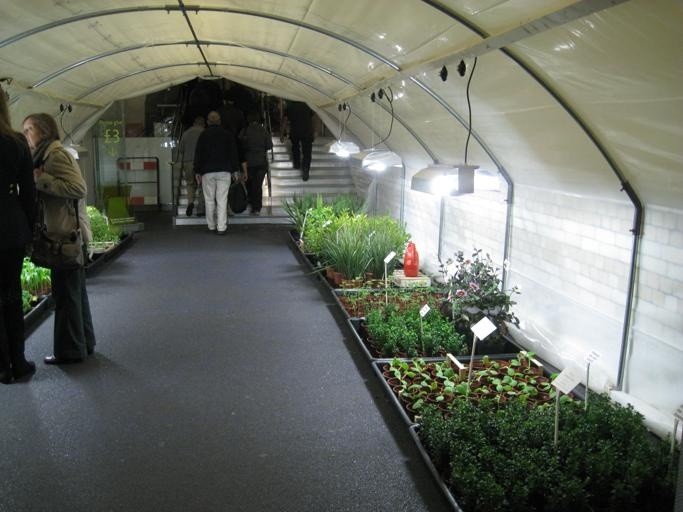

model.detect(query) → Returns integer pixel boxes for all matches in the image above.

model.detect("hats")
[208,111,221,122]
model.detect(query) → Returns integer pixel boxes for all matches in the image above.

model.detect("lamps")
[328,100,359,161]
[413,57,484,201]
[361,84,411,174]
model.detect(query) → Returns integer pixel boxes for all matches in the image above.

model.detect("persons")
[0,82,38,384]
[185,80,258,133]
[191,111,249,236]
[20,113,97,366]
[279,102,315,181]
[176,116,206,217]
[237,108,274,214]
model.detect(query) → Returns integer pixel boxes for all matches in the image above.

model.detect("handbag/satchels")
[29,197,84,270]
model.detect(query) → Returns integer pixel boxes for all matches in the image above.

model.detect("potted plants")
[317,215,409,289]
[357,300,469,358]
[337,275,444,316]
[381,350,575,421]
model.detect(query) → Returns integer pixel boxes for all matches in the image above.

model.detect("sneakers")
[251,209,259,214]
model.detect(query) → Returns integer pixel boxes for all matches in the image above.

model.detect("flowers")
[440,254,526,351]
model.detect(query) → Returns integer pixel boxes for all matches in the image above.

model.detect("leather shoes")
[197,212,206,217]
[15,360,35,377]
[185,202,194,216]
[216,228,228,235]
[44,355,83,363]
[208,229,217,233]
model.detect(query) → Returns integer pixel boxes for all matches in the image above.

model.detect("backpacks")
[229,181,249,214]
